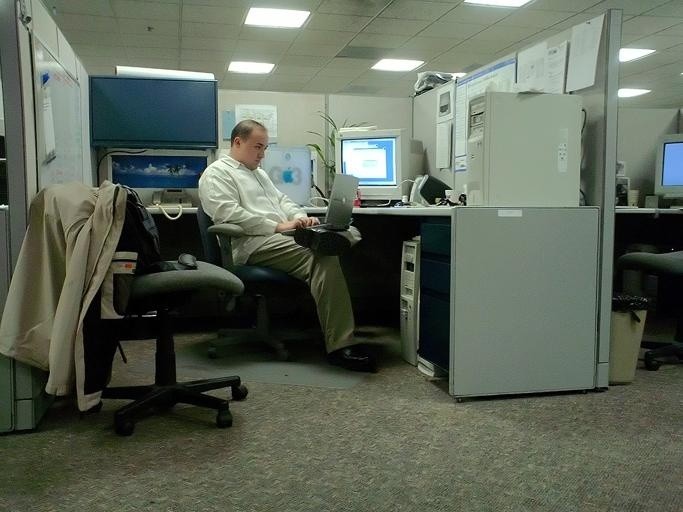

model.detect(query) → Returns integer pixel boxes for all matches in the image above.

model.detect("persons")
[199,120,382,371]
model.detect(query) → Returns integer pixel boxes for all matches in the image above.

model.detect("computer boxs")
[399,238,421,367]
[260,147,313,208]
[466,93,583,206]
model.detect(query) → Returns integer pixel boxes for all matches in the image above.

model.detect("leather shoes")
[326,348,371,371]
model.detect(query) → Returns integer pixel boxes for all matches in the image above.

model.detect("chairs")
[617,241,682,371]
[80,184,248,438]
[193,198,312,363]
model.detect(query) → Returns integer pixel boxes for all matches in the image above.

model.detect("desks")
[72,200,601,403]
[614,207,682,214]
[122,195,295,252]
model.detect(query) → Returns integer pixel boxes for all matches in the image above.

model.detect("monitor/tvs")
[334,129,425,207]
[654,133,683,208]
[89,74,219,149]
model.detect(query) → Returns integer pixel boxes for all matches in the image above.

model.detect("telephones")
[150,186,193,220]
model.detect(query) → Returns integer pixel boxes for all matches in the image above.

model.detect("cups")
[445,190,458,205]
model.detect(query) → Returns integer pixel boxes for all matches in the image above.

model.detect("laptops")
[282,173,360,236]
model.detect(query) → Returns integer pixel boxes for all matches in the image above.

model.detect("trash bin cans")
[608,296,651,385]
